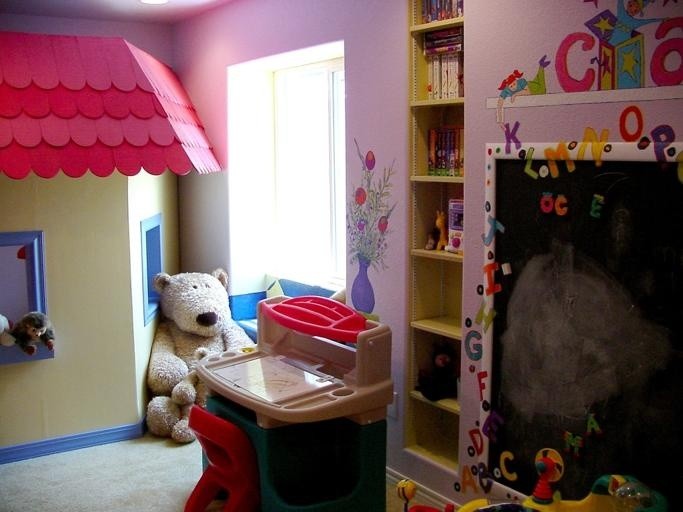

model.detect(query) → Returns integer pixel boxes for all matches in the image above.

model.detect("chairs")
[186,403,261,510]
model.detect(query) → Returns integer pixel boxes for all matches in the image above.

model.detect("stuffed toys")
[416,342,458,403]
[0,314,16,347]
[170,346,219,444]
[145,268,256,436]
[8,310,53,356]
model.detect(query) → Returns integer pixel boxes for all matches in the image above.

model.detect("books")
[417,0,463,177]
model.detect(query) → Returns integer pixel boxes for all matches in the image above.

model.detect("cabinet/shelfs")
[402,1,465,482]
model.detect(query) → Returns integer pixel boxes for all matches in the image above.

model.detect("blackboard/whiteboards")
[476,143,683,512]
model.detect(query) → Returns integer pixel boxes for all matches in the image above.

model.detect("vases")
[350,254,375,314]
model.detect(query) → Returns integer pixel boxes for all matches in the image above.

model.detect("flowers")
[344,137,398,273]
